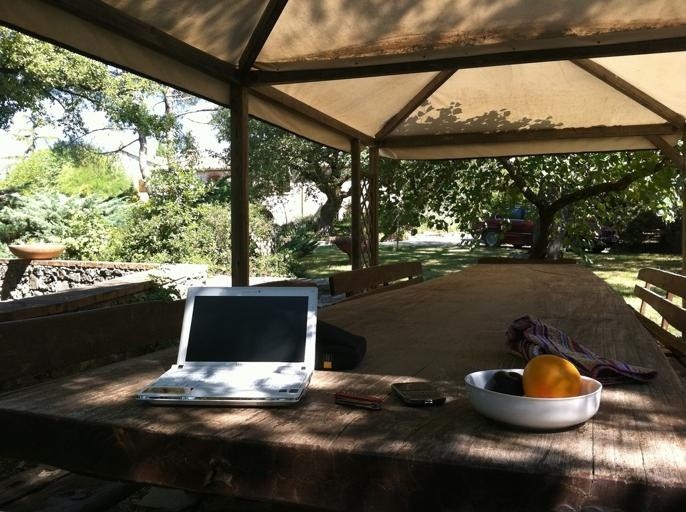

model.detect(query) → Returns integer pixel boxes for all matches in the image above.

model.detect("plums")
[484,370,524,396]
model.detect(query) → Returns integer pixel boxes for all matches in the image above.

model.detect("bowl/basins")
[464,368,603,430]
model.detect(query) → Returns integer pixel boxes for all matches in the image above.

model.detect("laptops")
[136,286,318,406]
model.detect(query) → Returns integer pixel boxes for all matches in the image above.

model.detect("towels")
[505,312,657,387]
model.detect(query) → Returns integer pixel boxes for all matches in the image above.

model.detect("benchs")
[328,261,425,307]
[0,281,317,511]
[627,268,686,393]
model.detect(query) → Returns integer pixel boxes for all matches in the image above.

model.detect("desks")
[0,258,686,512]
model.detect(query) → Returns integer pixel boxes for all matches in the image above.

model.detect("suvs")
[473,201,622,254]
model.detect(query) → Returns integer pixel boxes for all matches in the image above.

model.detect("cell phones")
[391,382,444,406]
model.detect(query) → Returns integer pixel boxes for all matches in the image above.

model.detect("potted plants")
[0,208,66,260]
